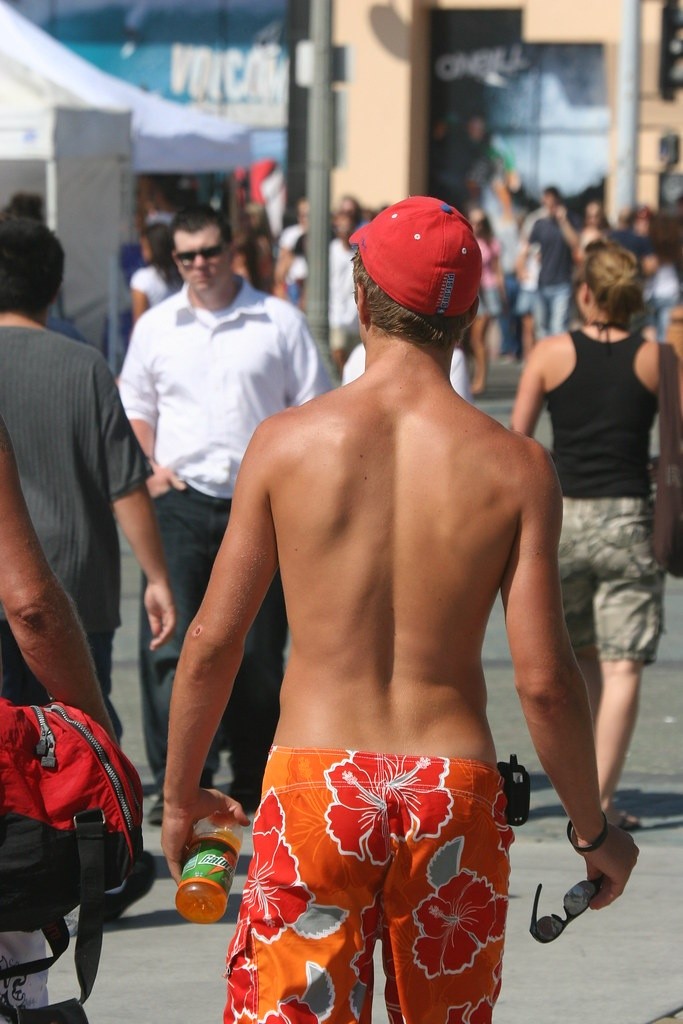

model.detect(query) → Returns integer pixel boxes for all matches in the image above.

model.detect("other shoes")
[612,811,641,830]
[104,850,155,921]
[149,779,212,825]
[229,779,260,811]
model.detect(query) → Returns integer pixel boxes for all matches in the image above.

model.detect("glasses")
[176,245,223,260]
[529,873,606,944]
[582,233,608,253]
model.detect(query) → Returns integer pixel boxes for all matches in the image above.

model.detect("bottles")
[175,811,244,924]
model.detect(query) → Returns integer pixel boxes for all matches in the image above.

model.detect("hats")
[349,195,482,317]
[468,207,486,223]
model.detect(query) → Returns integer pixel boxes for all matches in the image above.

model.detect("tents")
[2,0,254,375]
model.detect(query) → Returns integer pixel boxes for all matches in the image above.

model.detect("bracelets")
[567,812,608,851]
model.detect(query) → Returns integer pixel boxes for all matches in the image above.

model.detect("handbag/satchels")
[649,342,683,577]
[0,696,143,934]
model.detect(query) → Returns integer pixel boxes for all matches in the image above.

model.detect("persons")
[0,173,683,1024]
[159,197,640,1024]
[509,247,683,832]
